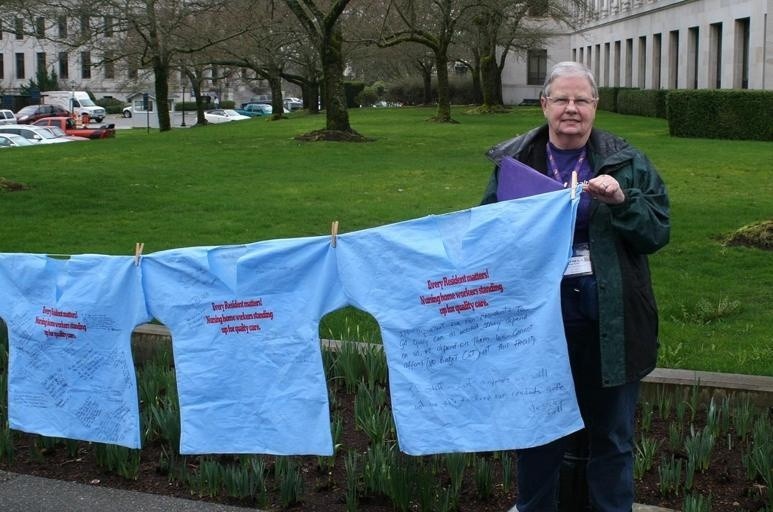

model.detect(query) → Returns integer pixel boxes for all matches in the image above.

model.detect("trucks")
[39,89,107,124]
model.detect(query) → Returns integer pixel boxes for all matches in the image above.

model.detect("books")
[495,154,563,204]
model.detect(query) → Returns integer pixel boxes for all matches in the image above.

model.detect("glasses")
[546,96,596,108]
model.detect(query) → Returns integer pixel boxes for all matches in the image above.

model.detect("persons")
[478,60,670,512]
[213,96,218,110]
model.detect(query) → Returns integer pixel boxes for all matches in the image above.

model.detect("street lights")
[179,78,189,126]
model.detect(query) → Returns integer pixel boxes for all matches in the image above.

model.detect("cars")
[0,103,116,149]
[231,97,305,117]
[123,105,144,117]
[359,100,404,108]
[203,107,251,123]
